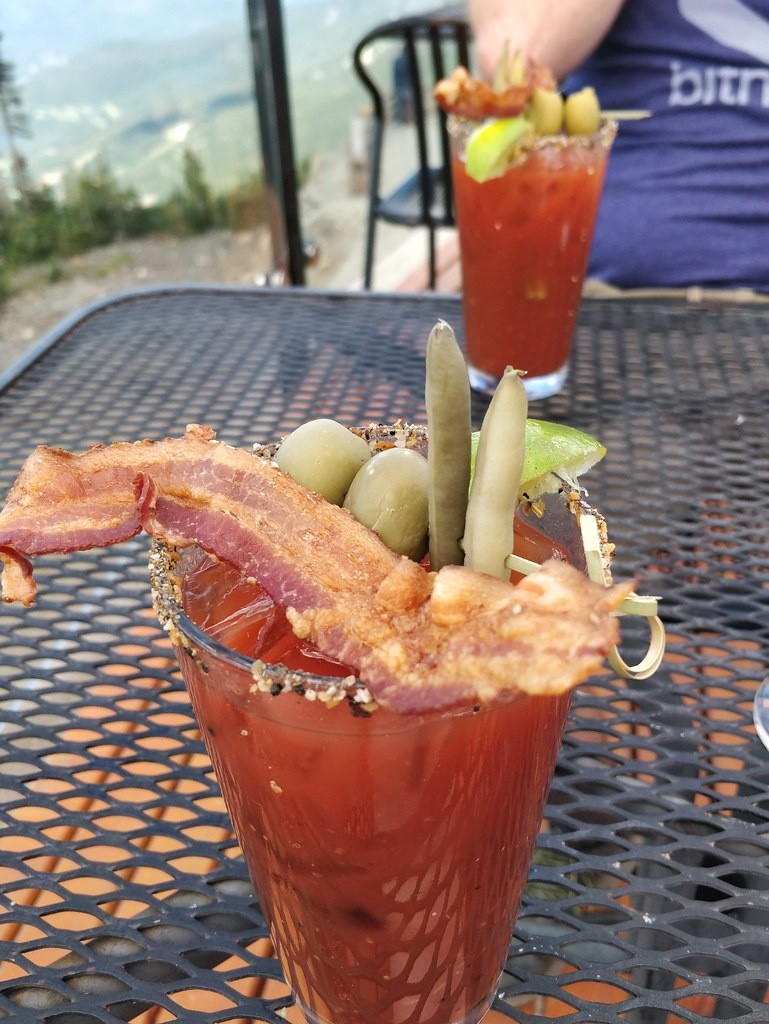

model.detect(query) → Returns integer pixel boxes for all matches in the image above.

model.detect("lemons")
[466,416,607,505]
[464,117,535,184]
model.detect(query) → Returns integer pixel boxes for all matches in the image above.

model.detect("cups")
[148,439,593,1024]
[446,113,617,401]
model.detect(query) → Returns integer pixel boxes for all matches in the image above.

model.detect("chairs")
[358,15,473,291]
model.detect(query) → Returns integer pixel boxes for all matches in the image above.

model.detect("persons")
[464,0,769,301]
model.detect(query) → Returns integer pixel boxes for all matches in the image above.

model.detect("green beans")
[425,318,528,580]
[494,40,524,92]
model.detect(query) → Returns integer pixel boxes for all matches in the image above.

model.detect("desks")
[0,287,769,1024]
